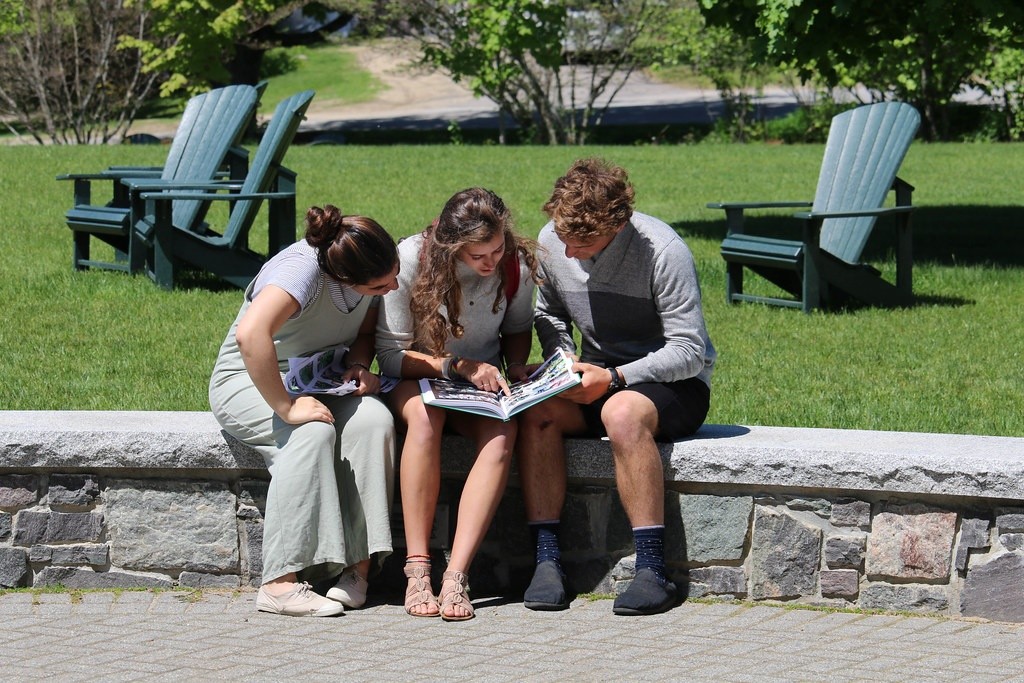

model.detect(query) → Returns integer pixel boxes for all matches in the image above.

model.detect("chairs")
[706,102,922,311]
[56,81,315,291]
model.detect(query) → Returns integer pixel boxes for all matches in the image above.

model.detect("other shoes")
[612,568,679,614]
[523,561,568,610]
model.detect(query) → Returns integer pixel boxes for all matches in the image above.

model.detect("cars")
[523,0,629,66]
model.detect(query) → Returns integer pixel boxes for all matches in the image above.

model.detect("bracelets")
[508,360,525,368]
[448,356,464,380]
[442,357,454,380]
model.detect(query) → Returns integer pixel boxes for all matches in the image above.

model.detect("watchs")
[607,368,624,393]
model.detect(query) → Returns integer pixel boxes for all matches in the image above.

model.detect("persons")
[375,189,542,622]
[517,157,717,615]
[208,204,400,617]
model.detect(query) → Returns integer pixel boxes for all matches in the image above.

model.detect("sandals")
[436,572,474,620]
[404,564,442,616]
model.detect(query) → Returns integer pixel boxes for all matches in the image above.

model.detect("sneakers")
[326,565,368,608]
[256,581,344,617]
